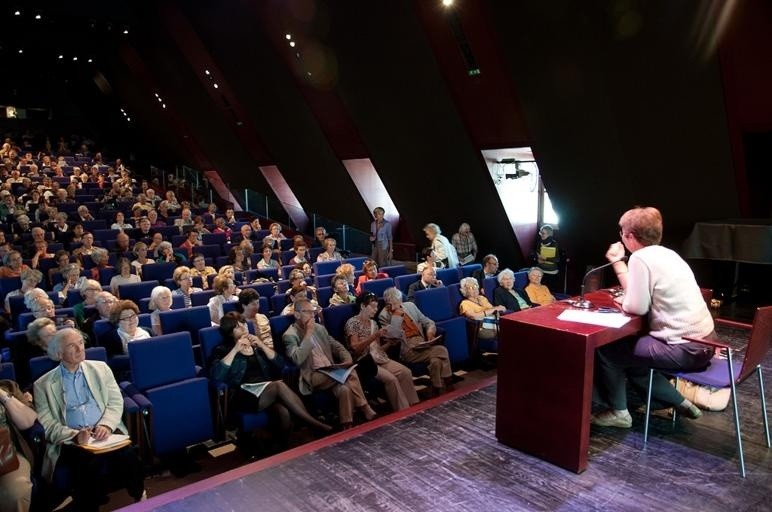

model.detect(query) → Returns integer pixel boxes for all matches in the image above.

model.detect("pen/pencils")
[79,424,94,434]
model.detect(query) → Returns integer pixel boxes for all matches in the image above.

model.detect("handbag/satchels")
[435,257,449,268]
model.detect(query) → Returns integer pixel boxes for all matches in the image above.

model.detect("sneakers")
[669,405,702,419]
[592,411,632,428]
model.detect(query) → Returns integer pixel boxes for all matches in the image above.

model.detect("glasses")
[119,314,136,321]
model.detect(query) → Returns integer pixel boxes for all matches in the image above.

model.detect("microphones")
[572,255,628,308]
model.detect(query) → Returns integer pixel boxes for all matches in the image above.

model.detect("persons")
[616,270,628,275]
[589,207,718,428]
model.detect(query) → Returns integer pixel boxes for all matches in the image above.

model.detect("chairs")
[0,144,563,512]
[639,305,771,481]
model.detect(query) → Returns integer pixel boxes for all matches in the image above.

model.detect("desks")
[496,286,718,476]
